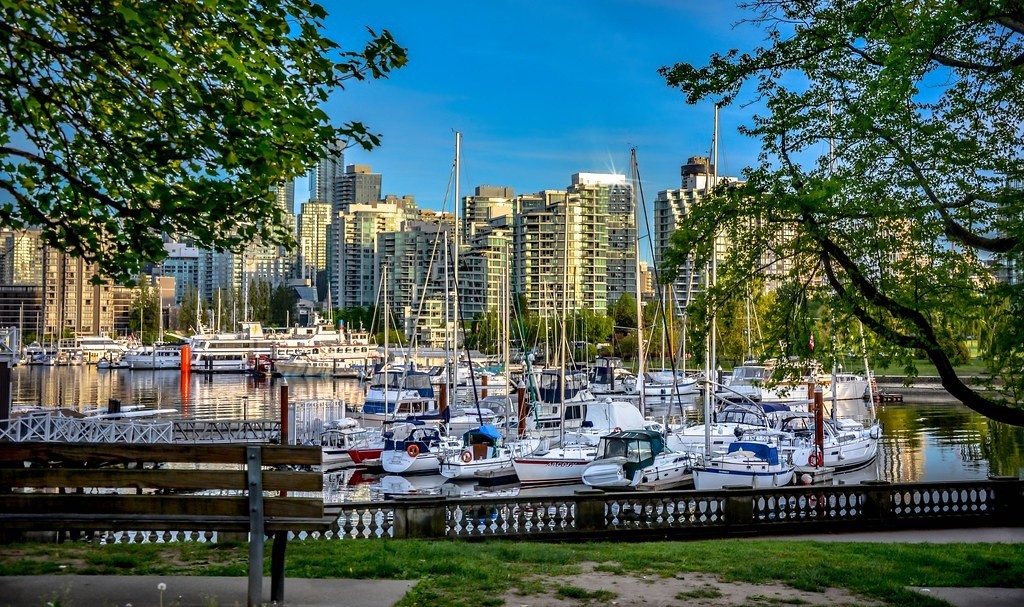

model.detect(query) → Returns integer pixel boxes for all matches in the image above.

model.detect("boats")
[21,265,392,380]
[368,475,460,498]
[582,428,700,490]
[307,416,382,474]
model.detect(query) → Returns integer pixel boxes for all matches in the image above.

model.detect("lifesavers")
[408,445,420,457]
[614,427,623,432]
[807,454,821,467]
[461,450,472,463]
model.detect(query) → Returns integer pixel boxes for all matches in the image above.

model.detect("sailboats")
[304,96,883,489]
